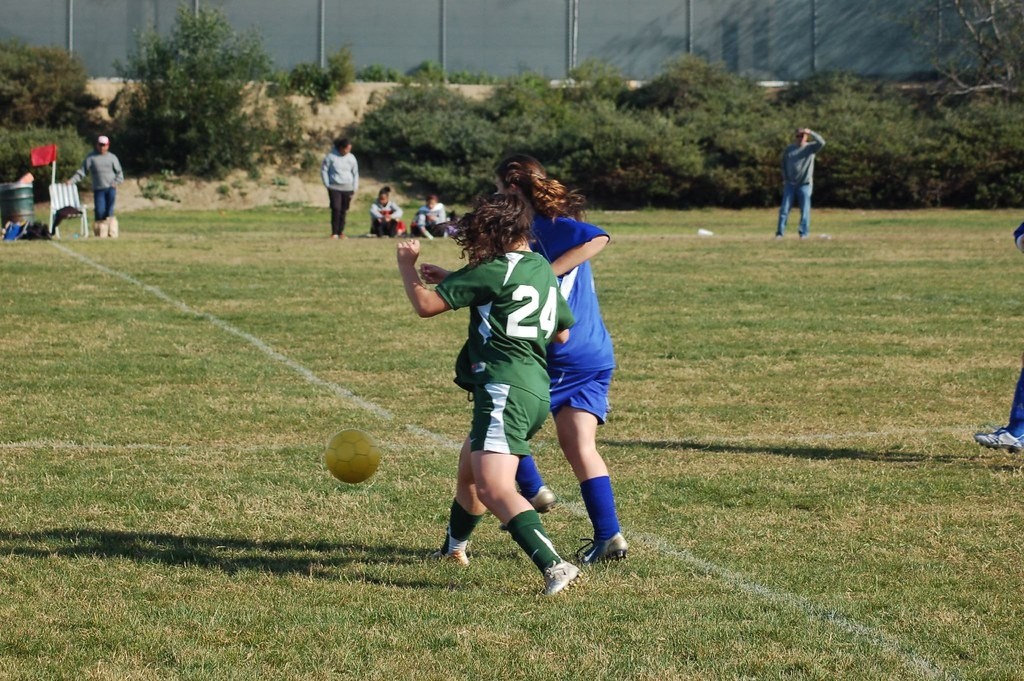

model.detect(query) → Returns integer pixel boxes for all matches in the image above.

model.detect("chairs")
[49,182,89,238]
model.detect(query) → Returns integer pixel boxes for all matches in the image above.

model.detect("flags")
[30,144,56,166]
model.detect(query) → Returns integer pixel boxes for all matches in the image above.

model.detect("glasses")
[98,144,107,147]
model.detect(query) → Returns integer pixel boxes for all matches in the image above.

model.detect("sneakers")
[433,550,469,565]
[974,427,1024,451]
[542,559,579,595]
[499,487,558,531]
[575,532,629,566]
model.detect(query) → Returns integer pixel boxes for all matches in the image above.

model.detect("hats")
[98,135,109,145]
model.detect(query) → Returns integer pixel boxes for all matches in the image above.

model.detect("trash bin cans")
[0,183,35,232]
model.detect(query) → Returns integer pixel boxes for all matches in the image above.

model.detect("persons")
[420,153,629,564]
[973,222,1024,452]
[410,196,448,239]
[362,186,403,238]
[320,138,359,241]
[775,127,826,240]
[396,194,579,596]
[64,135,124,221]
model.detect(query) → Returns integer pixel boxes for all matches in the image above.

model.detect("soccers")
[323,428,383,484]
